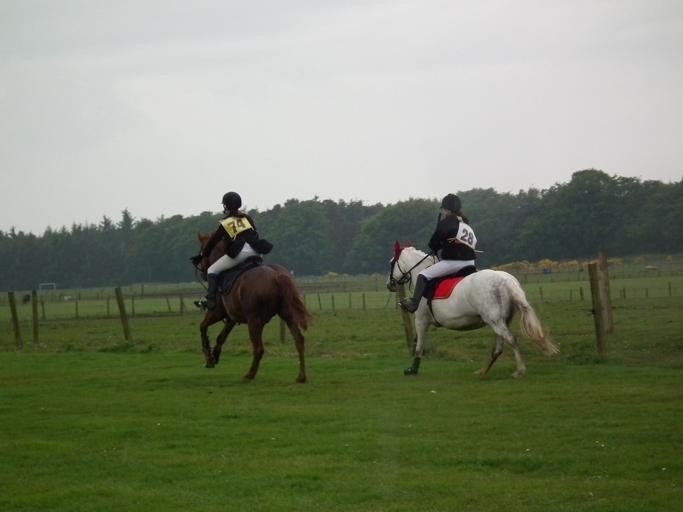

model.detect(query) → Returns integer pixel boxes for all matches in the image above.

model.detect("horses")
[198,233,315,383]
[386,240,561,380]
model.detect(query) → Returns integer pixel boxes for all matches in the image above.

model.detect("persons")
[397,194,478,312]
[194,192,274,309]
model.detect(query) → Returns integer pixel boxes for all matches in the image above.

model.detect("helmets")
[441,194,462,212]
[222,191,242,208]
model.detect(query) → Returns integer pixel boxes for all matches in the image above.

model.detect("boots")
[400,274,427,313]
[194,273,217,309]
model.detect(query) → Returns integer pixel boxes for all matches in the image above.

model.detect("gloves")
[190,255,203,265]
